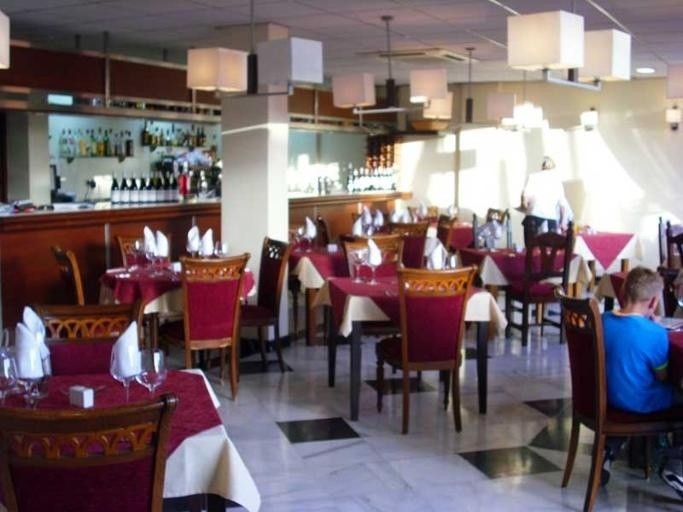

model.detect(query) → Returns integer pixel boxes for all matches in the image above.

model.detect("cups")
[109,348,140,407]
[135,346,168,404]
[14,346,44,414]
[0,345,17,410]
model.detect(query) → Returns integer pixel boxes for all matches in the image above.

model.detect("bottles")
[147,169,178,204]
[119,170,129,205]
[195,170,209,203]
[56,127,134,157]
[177,161,191,203]
[345,161,397,193]
[140,118,207,148]
[127,169,138,204]
[137,170,147,203]
[110,171,119,205]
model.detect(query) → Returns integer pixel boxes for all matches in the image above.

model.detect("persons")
[592,266,682,495]
[519,155,569,251]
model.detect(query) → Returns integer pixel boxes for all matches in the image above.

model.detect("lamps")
[246,0,257,94]
[506,2,631,93]
[332,14,448,113]
[382,15,395,106]
[186,0,324,99]
[464,45,473,122]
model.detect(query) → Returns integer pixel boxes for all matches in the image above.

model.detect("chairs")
[504,219,574,346]
[48,247,94,306]
[373,264,477,434]
[315,216,333,248]
[436,215,458,252]
[338,232,406,374]
[555,288,683,512]
[166,254,250,402]
[0,392,180,512]
[240,236,290,379]
[480,206,510,250]
[27,300,144,375]
[386,221,429,270]
[116,233,147,268]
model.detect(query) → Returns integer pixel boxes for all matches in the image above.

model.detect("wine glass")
[346,246,380,286]
[676,284,682,329]
[424,249,464,272]
[290,225,314,253]
[132,239,168,277]
[184,238,229,261]
[360,209,384,237]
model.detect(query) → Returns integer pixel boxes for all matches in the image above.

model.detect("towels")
[157,230,169,257]
[23,306,52,374]
[200,227,214,256]
[187,224,200,252]
[143,226,156,256]
[109,319,142,379]
[15,322,45,380]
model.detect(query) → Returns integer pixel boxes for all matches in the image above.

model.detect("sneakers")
[602,446,612,484]
[660,468,682,495]
